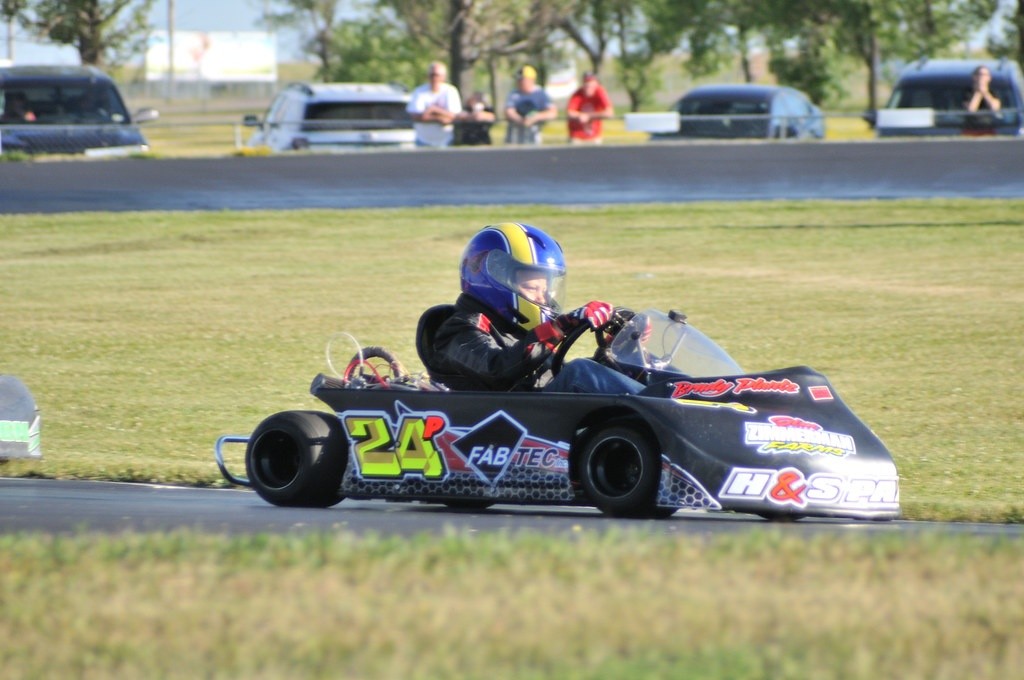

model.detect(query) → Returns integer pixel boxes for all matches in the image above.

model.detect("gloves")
[555,300,613,336]
[605,316,652,344]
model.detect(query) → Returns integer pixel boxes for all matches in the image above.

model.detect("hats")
[517,65,536,79]
[583,72,596,82]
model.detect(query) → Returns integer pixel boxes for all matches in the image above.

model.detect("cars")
[651,85,824,140]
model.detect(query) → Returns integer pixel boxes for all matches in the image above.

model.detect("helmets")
[460,222,567,340]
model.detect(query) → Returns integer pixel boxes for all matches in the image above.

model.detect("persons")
[416,223,694,395]
[9,92,38,121]
[566,72,614,144]
[506,66,556,143]
[407,61,497,147]
[960,64,1003,137]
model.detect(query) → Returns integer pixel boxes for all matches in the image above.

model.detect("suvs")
[1,62,158,158]
[862,54,1024,137]
[243,80,418,152]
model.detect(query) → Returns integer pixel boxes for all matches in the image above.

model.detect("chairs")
[416,304,537,393]
